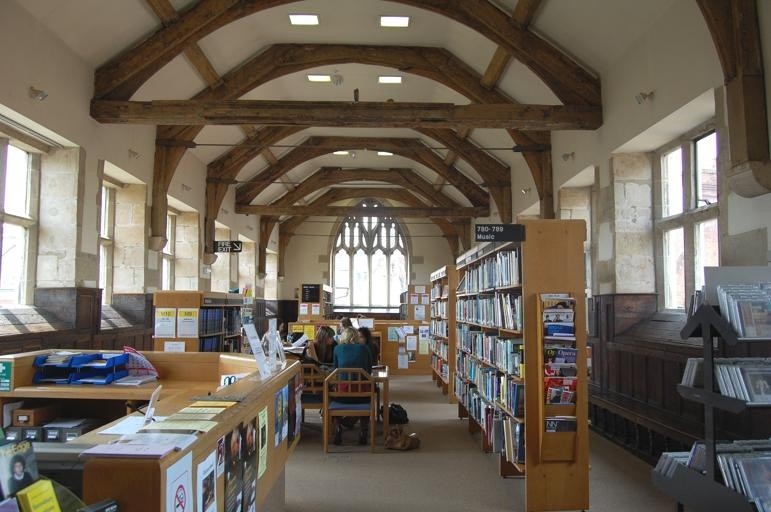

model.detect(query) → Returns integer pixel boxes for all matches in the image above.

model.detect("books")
[114,375,157,385]
[40,351,107,383]
[427,246,577,461]
[655,284,771,511]
[0,426,120,511]
[198,306,242,352]
[399,291,409,320]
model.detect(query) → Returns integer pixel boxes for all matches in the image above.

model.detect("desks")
[369,363,392,445]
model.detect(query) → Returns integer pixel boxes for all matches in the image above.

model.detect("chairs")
[282,340,379,455]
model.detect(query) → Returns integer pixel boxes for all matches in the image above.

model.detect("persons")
[357,326,381,366]
[300,325,338,367]
[330,326,375,445]
[339,316,354,331]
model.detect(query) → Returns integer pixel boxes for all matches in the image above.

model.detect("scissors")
[223,376,236,386]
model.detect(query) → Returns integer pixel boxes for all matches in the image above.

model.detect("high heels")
[332,425,344,447]
[357,431,367,445]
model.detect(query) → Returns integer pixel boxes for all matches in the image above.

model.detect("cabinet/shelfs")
[431,264,454,404]
[399,282,431,322]
[297,283,334,320]
[152,288,245,353]
[651,300,771,512]
[455,218,592,512]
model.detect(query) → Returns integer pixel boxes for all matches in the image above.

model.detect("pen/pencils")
[125,403,156,421]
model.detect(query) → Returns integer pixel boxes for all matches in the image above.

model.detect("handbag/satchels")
[380,403,411,423]
[383,426,421,451]
